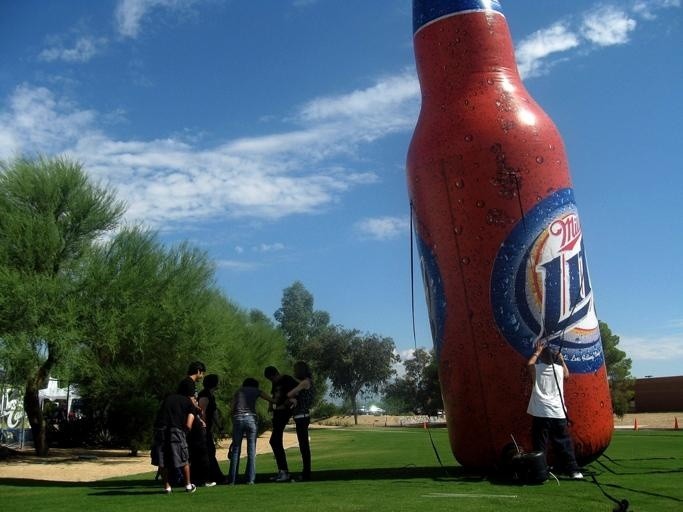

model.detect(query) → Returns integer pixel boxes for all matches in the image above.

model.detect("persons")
[263,364,301,482]
[41,399,85,427]
[177,361,217,488]
[286,360,316,481]
[222,377,277,486]
[196,374,230,484]
[150,394,202,494]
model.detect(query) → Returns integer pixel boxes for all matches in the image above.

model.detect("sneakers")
[205,482,216,487]
[186,484,196,493]
[165,489,173,495]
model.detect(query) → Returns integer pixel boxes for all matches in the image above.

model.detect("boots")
[270,470,290,482]
